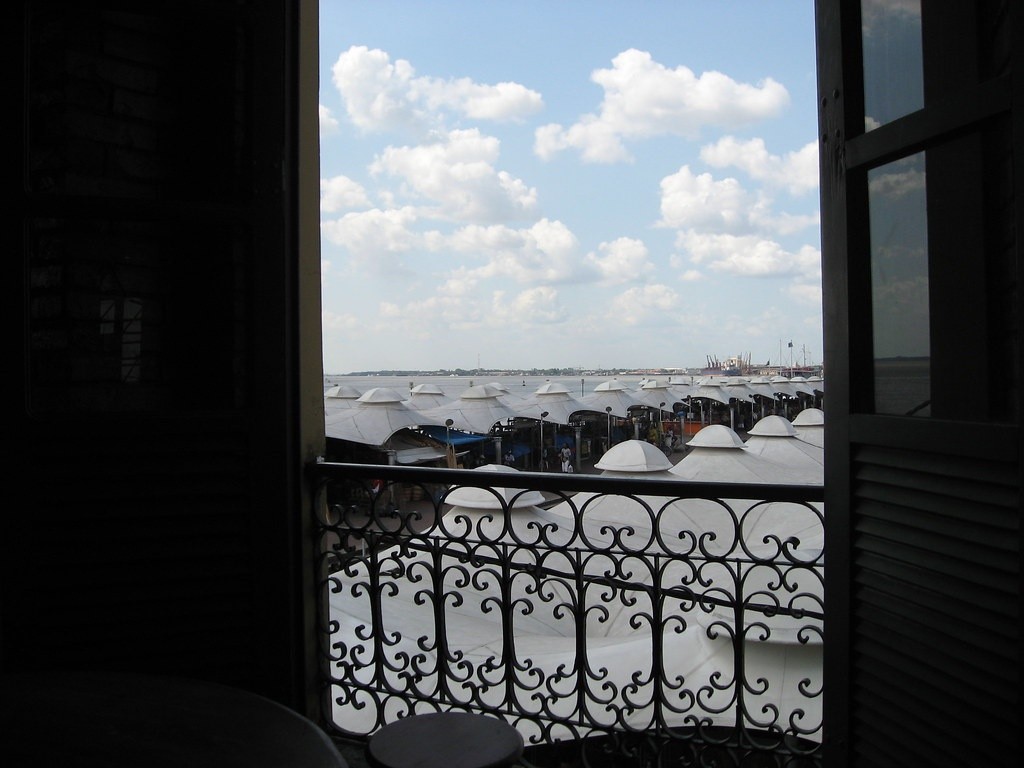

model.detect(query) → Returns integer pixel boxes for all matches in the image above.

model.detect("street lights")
[659,402,665,447]
[409,381,413,397]
[749,394,754,427]
[773,393,777,415]
[605,406,613,450]
[687,395,691,436]
[445,418,454,468]
[540,410,549,472]
[581,379,585,396]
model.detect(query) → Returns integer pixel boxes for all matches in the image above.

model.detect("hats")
[480,455,485,459]
[508,449,511,453]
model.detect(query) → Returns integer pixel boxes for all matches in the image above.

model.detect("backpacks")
[476,460,479,464]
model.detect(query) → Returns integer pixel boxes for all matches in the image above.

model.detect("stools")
[364,712,537,768]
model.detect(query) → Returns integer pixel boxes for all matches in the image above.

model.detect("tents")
[324,374,824,745]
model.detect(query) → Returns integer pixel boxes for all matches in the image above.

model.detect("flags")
[788,342,792,347]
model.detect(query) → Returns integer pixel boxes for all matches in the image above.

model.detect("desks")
[0,670,343,768]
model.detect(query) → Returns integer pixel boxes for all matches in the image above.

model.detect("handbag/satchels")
[568,465,573,473]
